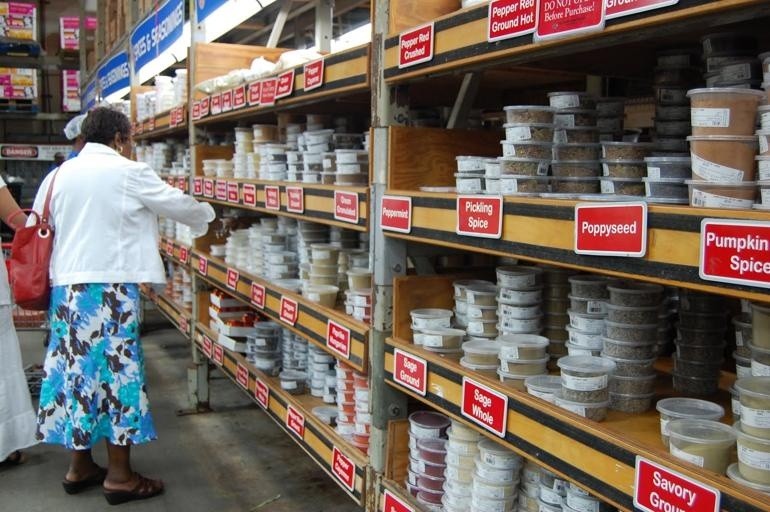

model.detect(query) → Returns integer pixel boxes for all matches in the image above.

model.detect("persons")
[19,105,251,507]
[0,167,47,471]
[34,112,95,203]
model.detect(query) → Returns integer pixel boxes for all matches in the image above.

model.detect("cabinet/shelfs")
[78,0,192,340]
[1,51,87,239]
[380,0,770,213]
[189,189,369,504]
[194,1,377,200]
[372,214,770,512]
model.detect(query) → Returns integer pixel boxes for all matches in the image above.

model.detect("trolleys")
[0,244,49,396]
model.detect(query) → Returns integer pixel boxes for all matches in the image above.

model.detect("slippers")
[63,462,107,495]
[103,471,165,506]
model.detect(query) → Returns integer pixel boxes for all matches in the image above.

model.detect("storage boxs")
[59,17,80,53]
[0,0,41,44]
[0,66,42,100]
[60,69,81,113]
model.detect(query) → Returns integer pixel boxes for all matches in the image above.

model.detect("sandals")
[0,451,24,469]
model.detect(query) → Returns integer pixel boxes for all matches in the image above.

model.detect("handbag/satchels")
[10,223,53,312]
